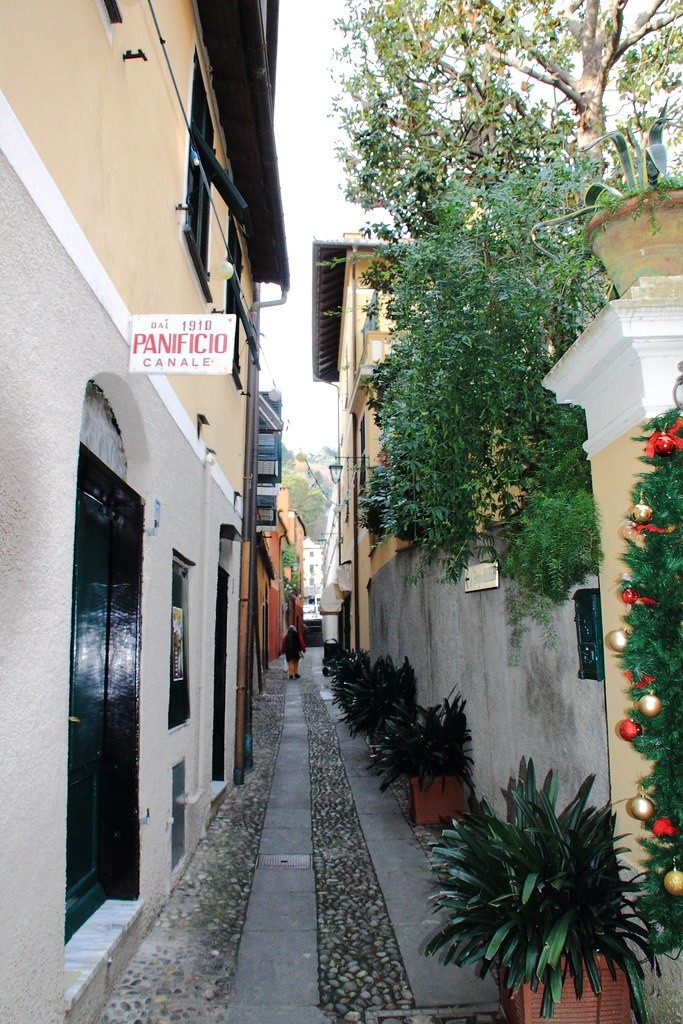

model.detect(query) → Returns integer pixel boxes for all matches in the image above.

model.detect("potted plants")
[417,753,665,1024]
[339,658,478,826]
[529,98,683,298]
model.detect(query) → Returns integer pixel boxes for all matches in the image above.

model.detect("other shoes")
[289,676,293,678]
[295,674,300,678]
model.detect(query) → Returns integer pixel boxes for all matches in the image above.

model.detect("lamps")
[240,391,251,397]
[296,448,307,462]
[329,455,370,484]
[207,262,235,280]
[191,145,199,166]
[123,49,147,62]
[269,382,281,401]
[325,497,332,507]
[333,505,339,513]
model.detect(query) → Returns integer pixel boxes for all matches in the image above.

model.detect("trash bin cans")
[324,638,337,658]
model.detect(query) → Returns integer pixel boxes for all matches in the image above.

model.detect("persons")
[282,625,306,678]
